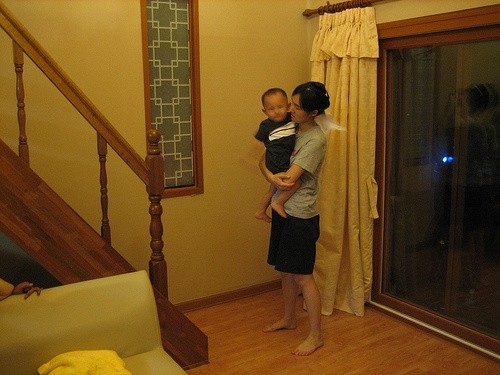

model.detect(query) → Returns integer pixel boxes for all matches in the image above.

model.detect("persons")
[254,87,301,223]
[0,278,43,300]
[259,81,331,355]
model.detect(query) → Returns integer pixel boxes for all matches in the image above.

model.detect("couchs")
[0,270,188,375]
